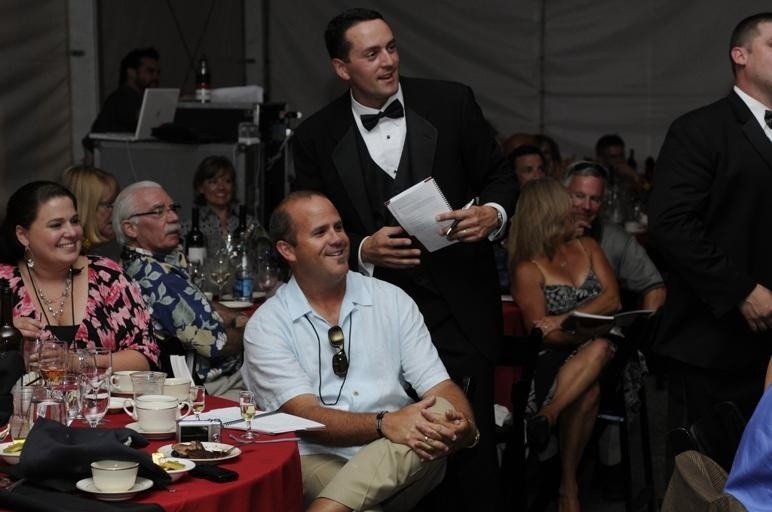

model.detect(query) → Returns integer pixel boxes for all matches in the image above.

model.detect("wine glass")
[23,338,112,430]
[190,386,206,420]
[239,391,258,440]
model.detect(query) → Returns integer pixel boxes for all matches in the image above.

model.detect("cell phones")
[189,464,239,482]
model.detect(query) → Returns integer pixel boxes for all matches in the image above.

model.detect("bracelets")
[231,311,242,327]
[93,352,98,366]
[374,410,388,436]
[465,430,480,450]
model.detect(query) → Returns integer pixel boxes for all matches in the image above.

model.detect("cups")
[108,370,192,432]
[194,58,212,102]
[185,251,279,308]
[91,459,139,491]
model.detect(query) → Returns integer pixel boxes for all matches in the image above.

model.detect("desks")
[95,138,266,227]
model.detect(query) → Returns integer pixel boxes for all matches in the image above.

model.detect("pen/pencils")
[446,199,475,236]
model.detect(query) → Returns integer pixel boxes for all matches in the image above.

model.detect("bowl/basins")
[625,222,641,230]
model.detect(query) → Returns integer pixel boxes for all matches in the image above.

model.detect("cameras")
[177,419,223,444]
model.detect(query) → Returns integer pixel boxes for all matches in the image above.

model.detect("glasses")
[328,325,347,377]
[127,204,182,221]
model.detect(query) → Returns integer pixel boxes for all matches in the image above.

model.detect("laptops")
[88,87,182,143]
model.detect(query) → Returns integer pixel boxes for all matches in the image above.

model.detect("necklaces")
[26,267,73,325]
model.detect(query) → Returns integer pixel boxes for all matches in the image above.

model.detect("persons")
[0,180,163,374]
[290,10,520,512]
[178,155,273,264]
[562,161,669,509]
[81,49,161,169]
[510,179,622,510]
[658,11,772,470]
[113,180,251,383]
[58,166,123,263]
[244,190,482,511]
[502,132,664,239]
[722,357,771,509]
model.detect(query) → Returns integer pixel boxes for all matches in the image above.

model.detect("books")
[559,309,655,334]
[384,176,463,254]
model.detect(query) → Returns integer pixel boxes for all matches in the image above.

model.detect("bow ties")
[360,98,404,132]
[765,111,772,127]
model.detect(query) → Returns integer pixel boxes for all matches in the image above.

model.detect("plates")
[0,442,21,465]
[150,441,242,481]
[76,477,154,501]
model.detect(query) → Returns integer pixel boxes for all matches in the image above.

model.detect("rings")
[544,324,548,330]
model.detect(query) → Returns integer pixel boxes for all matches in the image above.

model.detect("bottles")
[184,204,258,302]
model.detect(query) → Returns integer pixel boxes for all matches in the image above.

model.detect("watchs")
[492,206,503,227]
